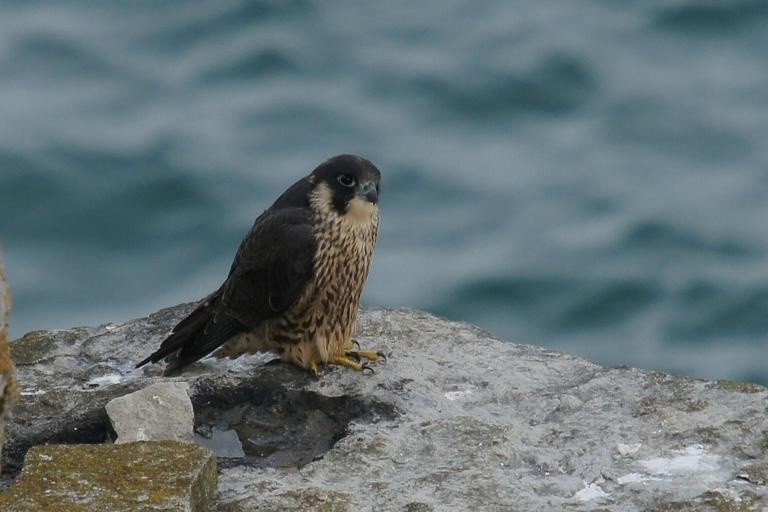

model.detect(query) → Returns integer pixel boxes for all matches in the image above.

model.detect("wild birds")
[133,153,385,382]
